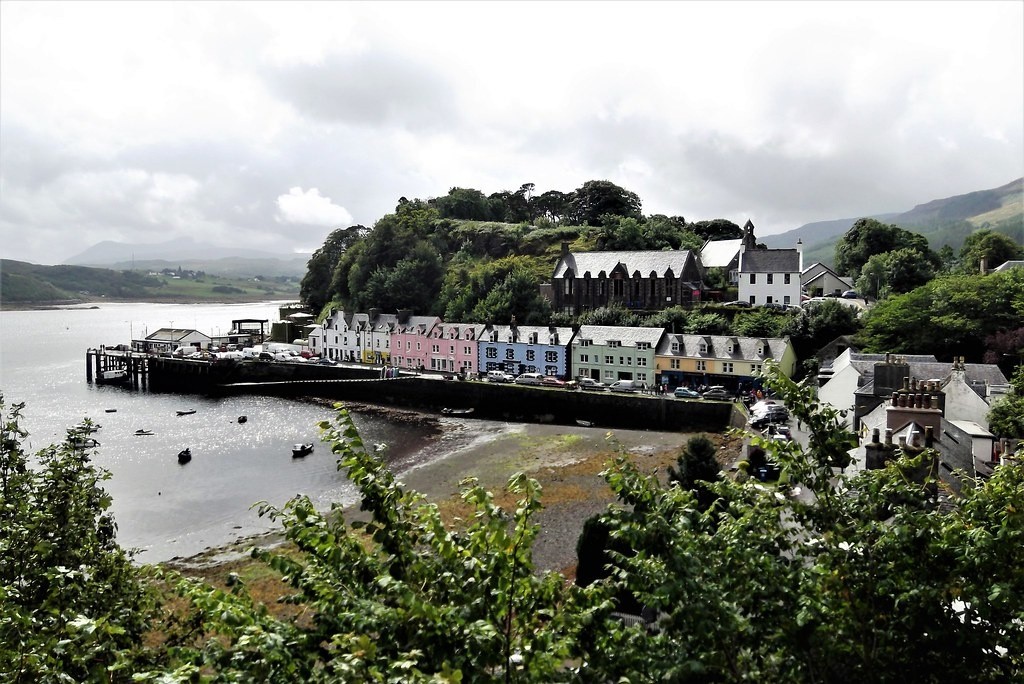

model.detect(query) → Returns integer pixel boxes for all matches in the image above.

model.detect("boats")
[178,448,191,465]
[105,408,117,413]
[135,429,154,435]
[176,408,196,416]
[292,443,314,458]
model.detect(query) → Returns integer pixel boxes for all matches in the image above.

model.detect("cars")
[320,358,337,366]
[259,349,307,364]
[674,386,700,399]
[198,344,242,361]
[543,377,566,388]
[308,357,319,364]
[301,350,313,359]
[750,400,789,430]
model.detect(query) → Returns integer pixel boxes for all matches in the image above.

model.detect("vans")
[610,380,636,393]
[515,373,544,386]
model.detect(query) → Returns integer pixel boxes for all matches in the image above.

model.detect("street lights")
[143,323,149,352]
[125,320,134,351]
[169,320,174,354]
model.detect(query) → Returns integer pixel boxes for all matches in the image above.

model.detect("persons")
[751,388,762,399]
[679,381,690,389]
[639,383,668,397]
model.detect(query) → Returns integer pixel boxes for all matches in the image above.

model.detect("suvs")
[487,371,514,382]
[580,378,605,390]
[703,389,729,401]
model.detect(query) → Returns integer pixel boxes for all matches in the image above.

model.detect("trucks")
[243,348,260,358]
[174,347,196,357]
